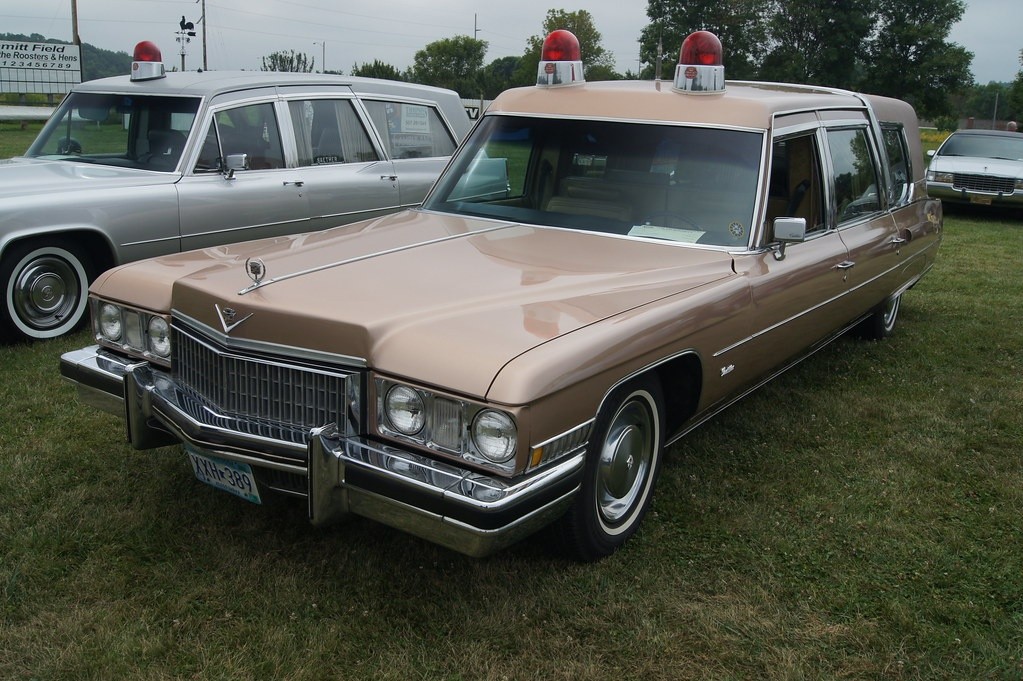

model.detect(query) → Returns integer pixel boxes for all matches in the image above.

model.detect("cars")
[926,128,1022,216]
[55,29,942,561]
[0,39,511,343]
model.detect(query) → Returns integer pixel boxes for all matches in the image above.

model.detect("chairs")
[318,127,343,162]
[223,128,266,169]
[543,168,788,244]
[148,129,187,167]
